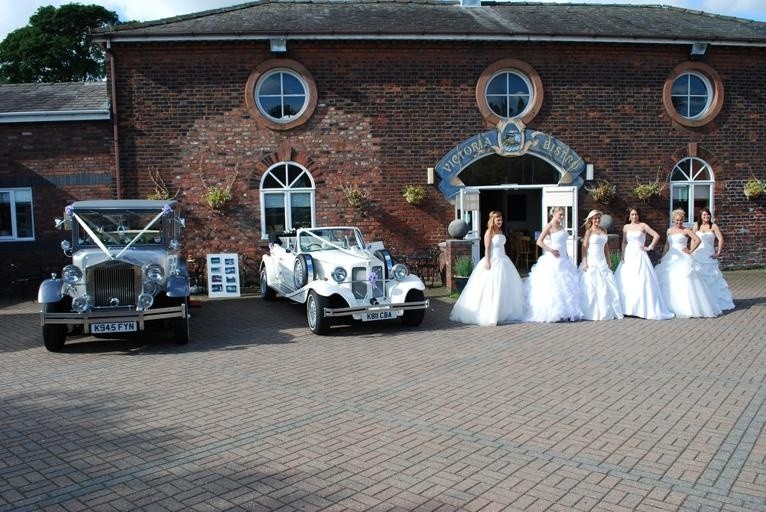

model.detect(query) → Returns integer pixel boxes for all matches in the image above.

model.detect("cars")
[37,199,195,349]
[259,225,430,336]
[0,254,54,300]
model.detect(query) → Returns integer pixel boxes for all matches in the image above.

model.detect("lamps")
[687,42,710,56]
[266,38,289,55]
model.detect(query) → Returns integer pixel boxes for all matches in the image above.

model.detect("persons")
[654,208,723,319]
[613,207,677,321]
[520,206,585,323]
[689,209,736,311]
[576,209,625,321]
[448,211,524,325]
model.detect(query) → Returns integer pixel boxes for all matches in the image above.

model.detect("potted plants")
[633,185,655,202]
[206,188,232,210]
[744,180,764,200]
[591,184,617,205]
[453,254,475,296]
[405,187,425,205]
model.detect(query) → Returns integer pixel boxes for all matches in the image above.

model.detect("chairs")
[515,236,535,270]
[391,248,442,288]
